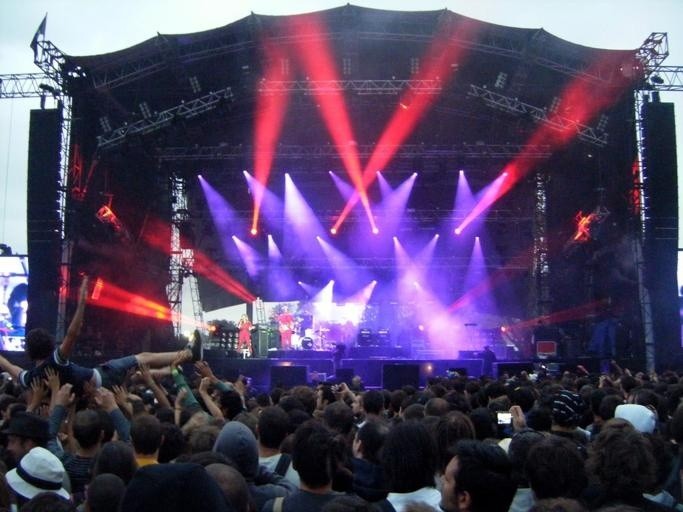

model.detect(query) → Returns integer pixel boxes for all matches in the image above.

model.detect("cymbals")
[321,329,331,333]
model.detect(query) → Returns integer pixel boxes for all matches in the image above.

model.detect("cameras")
[495,411,513,424]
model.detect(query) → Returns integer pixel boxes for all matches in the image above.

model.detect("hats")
[0,412,50,446]
[613,404,656,435]
[549,389,586,428]
[4,445,73,499]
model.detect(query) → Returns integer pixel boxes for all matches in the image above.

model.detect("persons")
[1,276,203,394]
[276,306,296,348]
[1,351,683,511]
[237,314,253,353]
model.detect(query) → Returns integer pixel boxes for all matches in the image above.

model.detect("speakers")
[269,365,306,389]
[640,101,678,294]
[26,108,65,295]
[381,363,419,390]
[493,362,530,382]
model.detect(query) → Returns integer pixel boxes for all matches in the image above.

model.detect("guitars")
[278,318,304,333]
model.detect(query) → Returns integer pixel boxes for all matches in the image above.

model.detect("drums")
[301,330,321,349]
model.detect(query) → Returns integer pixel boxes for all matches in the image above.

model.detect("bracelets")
[175,407,182,410]
[172,369,178,375]
